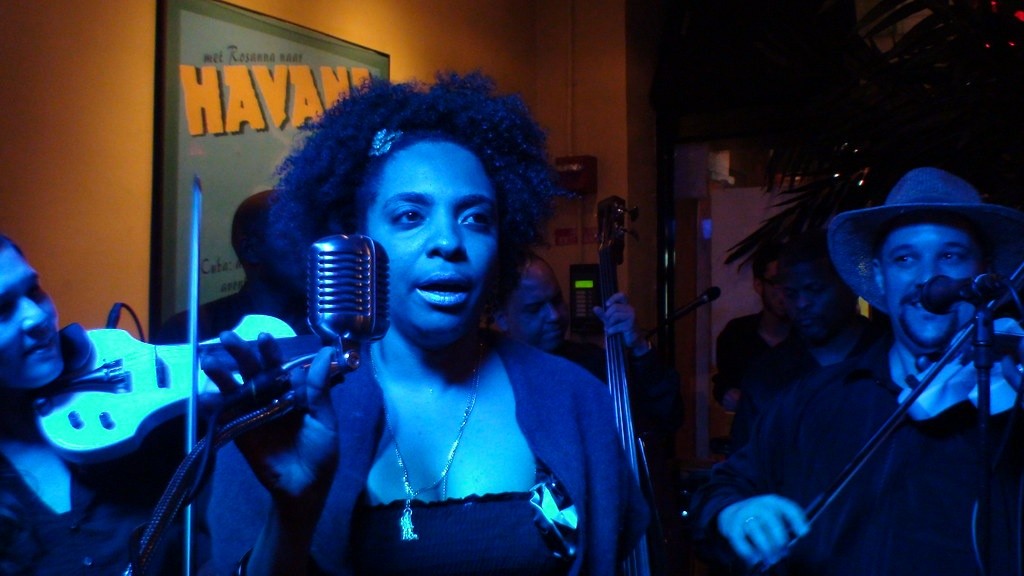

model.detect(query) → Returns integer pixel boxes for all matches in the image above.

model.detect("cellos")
[592,195,685,574]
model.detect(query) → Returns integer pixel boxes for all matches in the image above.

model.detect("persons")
[684,165,1023,576]
[487,251,684,576]
[153,193,309,345]
[169,68,650,576]
[0,233,178,576]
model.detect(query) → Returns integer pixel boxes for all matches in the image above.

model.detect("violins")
[898,317,1024,422]
[35,311,299,462]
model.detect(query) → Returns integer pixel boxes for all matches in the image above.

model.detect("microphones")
[920,274,1010,315]
[271,233,393,413]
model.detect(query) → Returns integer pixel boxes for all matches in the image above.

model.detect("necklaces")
[371,352,480,539]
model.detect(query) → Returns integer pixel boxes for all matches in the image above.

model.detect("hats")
[826,166,1024,316]
